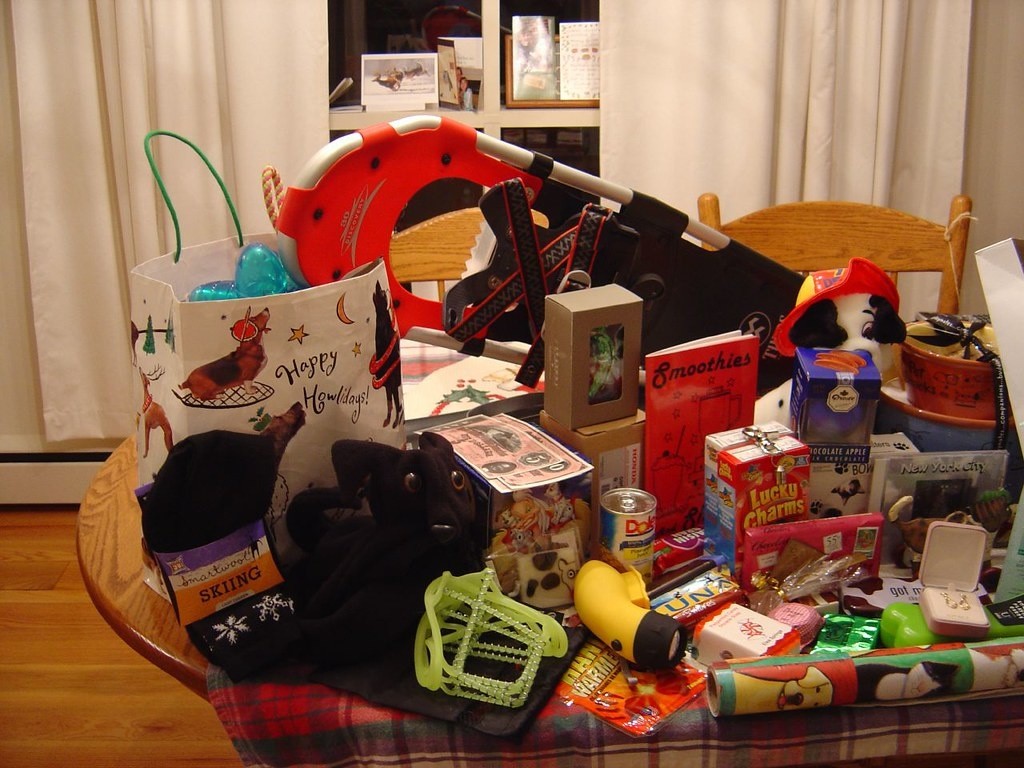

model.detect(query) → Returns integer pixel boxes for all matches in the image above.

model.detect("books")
[644,330,760,537]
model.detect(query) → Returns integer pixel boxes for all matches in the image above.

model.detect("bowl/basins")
[899,321,1012,421]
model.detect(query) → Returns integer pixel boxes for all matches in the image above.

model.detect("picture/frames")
[504,35,599,108]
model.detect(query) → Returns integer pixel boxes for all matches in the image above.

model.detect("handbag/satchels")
[127,131,407,578]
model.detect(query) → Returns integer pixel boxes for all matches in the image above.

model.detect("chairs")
[698,194,972,321]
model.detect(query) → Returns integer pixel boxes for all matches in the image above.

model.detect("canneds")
[600,487,656,586]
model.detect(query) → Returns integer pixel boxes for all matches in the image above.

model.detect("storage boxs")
[133,285,991,662]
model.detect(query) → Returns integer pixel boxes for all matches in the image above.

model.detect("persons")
[443,69,453,100]
[456,67,474,111]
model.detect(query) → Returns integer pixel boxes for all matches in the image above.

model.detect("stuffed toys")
[753,257,907,428]
[262,431,477,671]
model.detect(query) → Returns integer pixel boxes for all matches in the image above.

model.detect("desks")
[77,427,1024,768]
